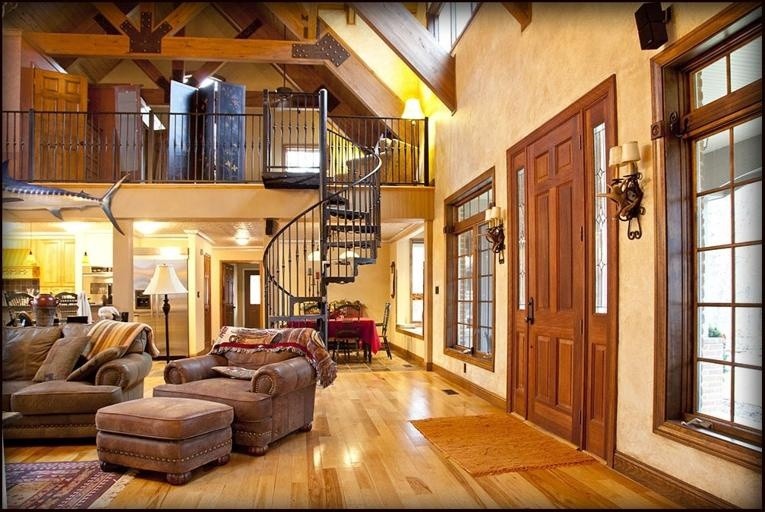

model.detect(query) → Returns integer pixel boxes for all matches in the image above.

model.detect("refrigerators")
[133,247,190,358]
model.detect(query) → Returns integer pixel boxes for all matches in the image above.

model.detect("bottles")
[34,293,60,307]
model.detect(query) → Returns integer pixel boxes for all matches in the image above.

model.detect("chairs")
[54,292,79,319]
[301,299,392,364]
[154,326,322,456]
[4,290,34,320]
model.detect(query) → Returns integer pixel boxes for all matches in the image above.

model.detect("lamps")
[484,206,505,265]
[143,263,188,365]
[233,226,254,248]
[81,251,89,265]
[305,242,362,262]
[273,25,292,102]
[594,140,647,240]
[23,223,37,267]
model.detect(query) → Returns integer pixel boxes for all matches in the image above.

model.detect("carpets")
[4,460,142,509]
[411,413,596,475]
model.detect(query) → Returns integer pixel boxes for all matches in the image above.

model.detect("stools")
[95,396,234,485]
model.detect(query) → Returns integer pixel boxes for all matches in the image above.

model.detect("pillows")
[31,335,92,382]
[65,345,126,382]
[379,138,392,152]
[211,365,257,380]
[2,326,63,381]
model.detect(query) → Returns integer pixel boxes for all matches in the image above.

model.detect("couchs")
[346,138,419,185]
[1,323,153,439]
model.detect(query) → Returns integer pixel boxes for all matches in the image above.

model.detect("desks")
[286,317,375,364]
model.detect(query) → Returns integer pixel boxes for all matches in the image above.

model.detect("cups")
[33,307,55,328]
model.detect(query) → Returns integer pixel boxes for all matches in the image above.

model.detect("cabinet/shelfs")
[20,60,246,183]
